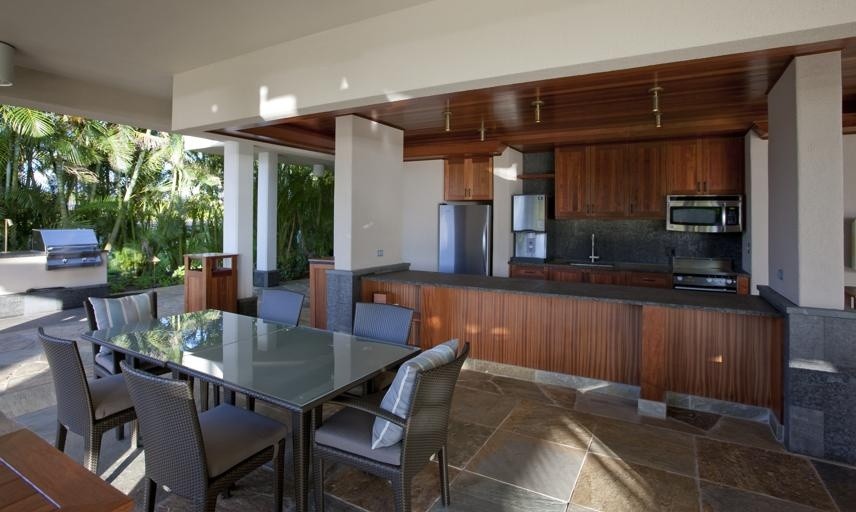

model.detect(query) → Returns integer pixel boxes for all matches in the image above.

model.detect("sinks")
[570,263,613,267]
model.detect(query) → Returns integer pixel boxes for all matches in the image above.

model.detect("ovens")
[666,194,742,233]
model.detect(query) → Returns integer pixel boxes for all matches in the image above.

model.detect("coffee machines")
[512,195,546,259]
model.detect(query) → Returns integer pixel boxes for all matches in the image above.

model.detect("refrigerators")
[437,203,490,274]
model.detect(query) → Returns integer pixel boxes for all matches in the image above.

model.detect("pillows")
[372,338,459,451]
[88,293,153,356]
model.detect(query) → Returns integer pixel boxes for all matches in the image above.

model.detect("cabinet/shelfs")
[554,137,745,219]
[508,264,581,282]
[444,157,493,200]
[588,270,672,288]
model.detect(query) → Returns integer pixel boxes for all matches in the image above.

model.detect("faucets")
[589,234,599,263]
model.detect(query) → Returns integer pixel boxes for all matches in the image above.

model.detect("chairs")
[310,341,470,512]
[246,290,305,413]
[83,291,158,442]
[119,359,289,512]
[335,302,414,400]
[37,326,139,475]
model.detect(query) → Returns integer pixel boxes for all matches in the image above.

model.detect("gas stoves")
[674,256,737,292]
